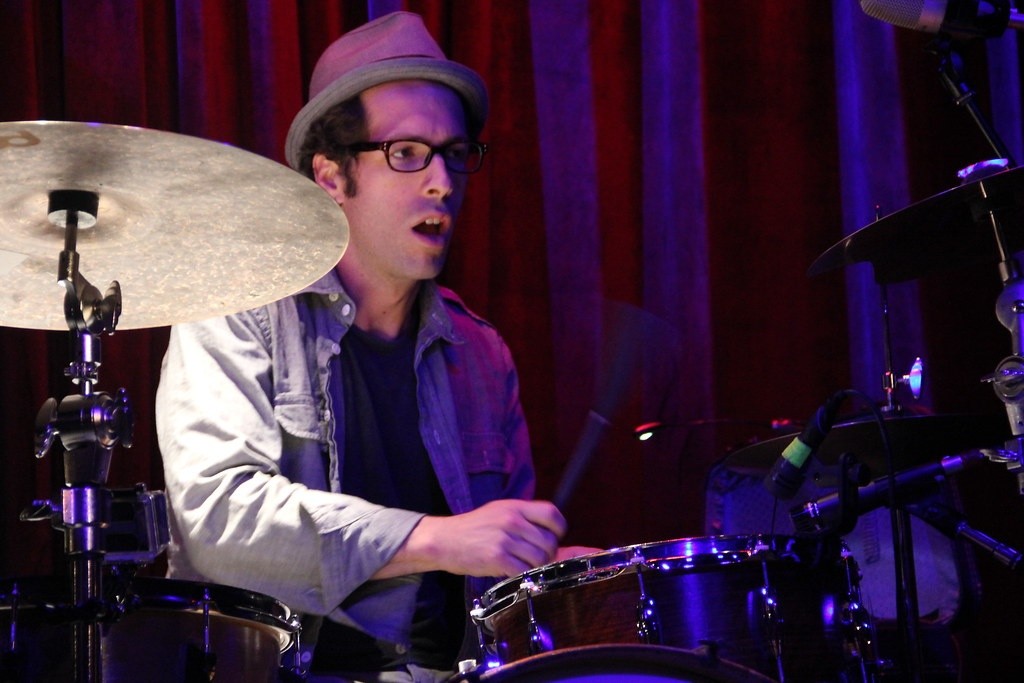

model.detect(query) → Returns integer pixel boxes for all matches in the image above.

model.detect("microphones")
[860,0,1024,39]
[788,450,985,539]
[764,391,848,499]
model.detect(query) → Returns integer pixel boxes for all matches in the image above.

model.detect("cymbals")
[814,163,1024,289]
[0,120,352,332]
[722,406,1006,474]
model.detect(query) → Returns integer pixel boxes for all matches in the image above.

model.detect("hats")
[283,11,489,181]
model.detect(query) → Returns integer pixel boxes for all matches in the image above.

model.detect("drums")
[469,533,879,683]
[1,576,300,683]
[435,641,778,683]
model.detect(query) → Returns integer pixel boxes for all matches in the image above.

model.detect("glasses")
[334,135,489,176]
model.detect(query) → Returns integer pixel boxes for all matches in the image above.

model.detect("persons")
[156,10,607,683]
